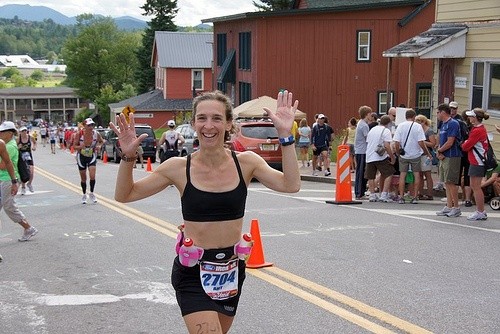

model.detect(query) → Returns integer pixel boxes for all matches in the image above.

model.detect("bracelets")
[279,135,296,146]
[120,152,140,161]
[13,179,18,184]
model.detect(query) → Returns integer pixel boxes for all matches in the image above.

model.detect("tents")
[232,95,306,124]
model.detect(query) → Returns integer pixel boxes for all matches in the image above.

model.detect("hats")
[0,121,18,135]
[318,113,327,119]
[466,109,477,117]
[84,118,95,125]
[19,126,27,132]
[449,101,459,109]
[167,120,176,127]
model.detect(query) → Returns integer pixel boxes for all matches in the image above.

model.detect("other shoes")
[17,226,39,241]
[356,189,434,204]
[465,201,472,207]
[324,170,331,176]
[89,193,98,203]
[467,209,488,221]
[433,183,445,191]
[21,189,26,195]
[436,206,452,216]
[27,182,34,192]
[312,171,318,176]
[446,207,462,217]
[81,194,87,204]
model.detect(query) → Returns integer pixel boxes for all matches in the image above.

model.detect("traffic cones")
[246,219,273,269]
[145,158,152,171]
[71,147,74,154]
[103,151,108,163]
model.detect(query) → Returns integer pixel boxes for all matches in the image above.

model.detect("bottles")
[238,234,253,260]
[423,156,430,167]
[178,238,199,267]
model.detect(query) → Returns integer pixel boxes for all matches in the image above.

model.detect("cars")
[158,124,199,157]
[227,117,283,181]
[100,125,158,163]
[96,128,107,158]
[32,119,44,126]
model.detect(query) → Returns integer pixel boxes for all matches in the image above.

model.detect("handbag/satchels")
[375,144,386,155]
[399,147,405,156]
[483,145,499,172]
[432,155,440,164]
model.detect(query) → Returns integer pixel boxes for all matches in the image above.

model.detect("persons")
[292,100,500,220]
[0,117,199,264]
[110,89,301,334]
